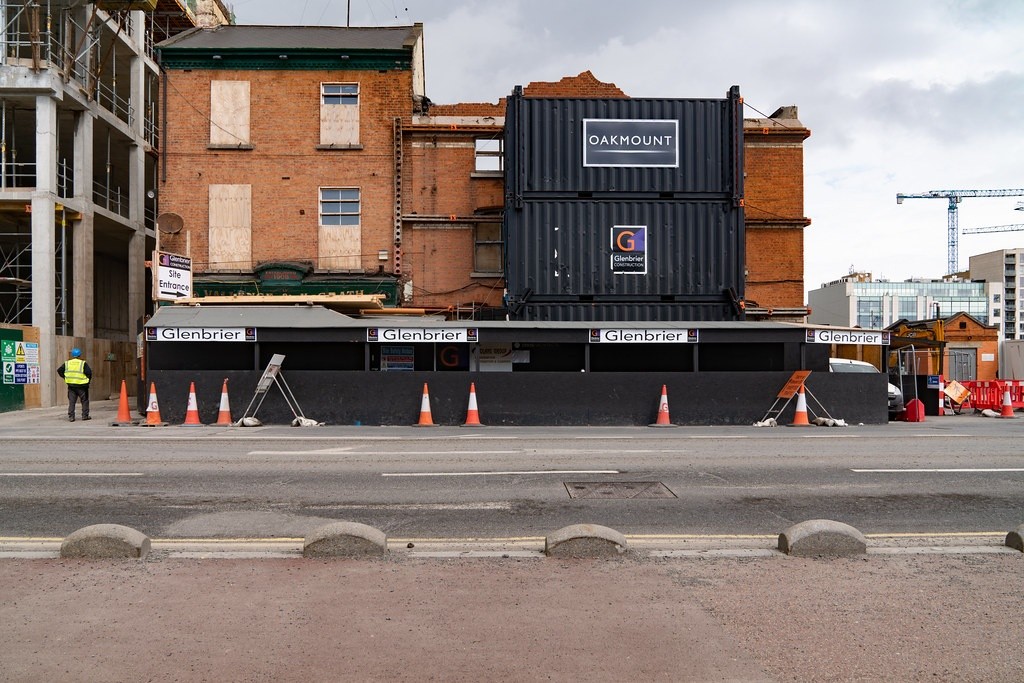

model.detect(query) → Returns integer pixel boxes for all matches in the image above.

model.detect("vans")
[827,358,904,419]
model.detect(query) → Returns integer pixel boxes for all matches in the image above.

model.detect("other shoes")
[69,418,76,422]
[82,416,91,420]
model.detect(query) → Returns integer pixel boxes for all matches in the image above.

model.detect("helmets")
[71,348,82,357]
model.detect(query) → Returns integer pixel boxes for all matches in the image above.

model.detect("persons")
[57,348,92,422]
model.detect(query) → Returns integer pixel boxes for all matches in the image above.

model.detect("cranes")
[962,200,1024,231]
[897,188,1024,276]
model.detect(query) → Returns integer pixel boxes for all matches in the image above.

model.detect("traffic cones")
[209,378,235,425]
[648,384,678,428]
[996,383,1018,418]
[412,382,440,427]
[108,380,138,428]
[138,381,169,427]
[179,382,207,427]
[786,383,815,427]
[458,381,486,427]
